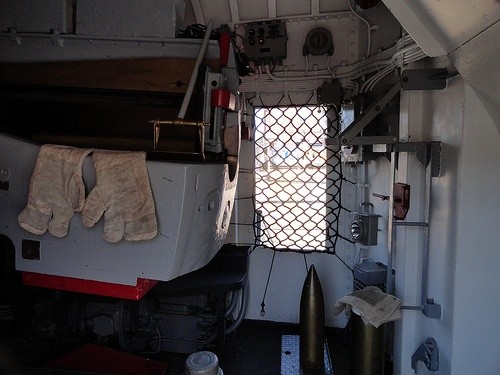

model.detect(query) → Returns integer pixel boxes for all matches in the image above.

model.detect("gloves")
[18,144,93,237]
[82,150,157,243]
